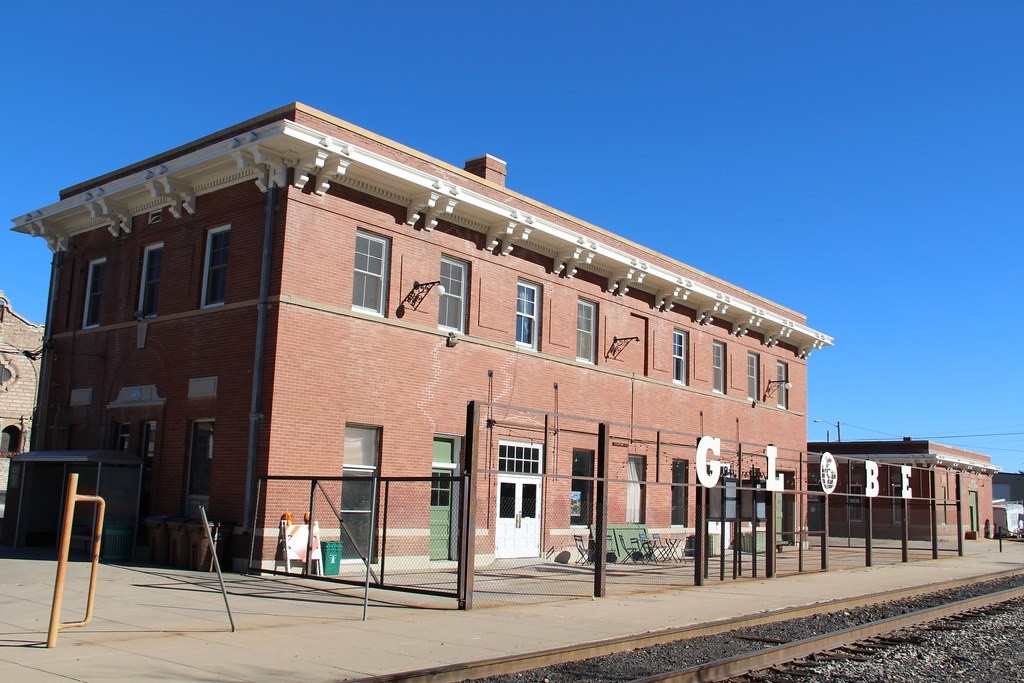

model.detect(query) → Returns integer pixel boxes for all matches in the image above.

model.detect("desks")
[661,536,686,563]
[637,538,660,565]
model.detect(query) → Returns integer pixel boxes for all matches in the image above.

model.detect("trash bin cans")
[103,519,136,560]
[166,517,190,567]
[321,541,343,575]
[144,515,171,564]
[185,519,236,571]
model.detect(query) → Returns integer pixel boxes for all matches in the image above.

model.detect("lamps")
[406,280,445,311]
[767,379,792,398]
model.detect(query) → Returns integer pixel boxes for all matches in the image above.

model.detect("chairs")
[652,534,672,561]
[574,534,595,566]
[681,537,695,563]
[606,535,616,553]
[639,533,660,562]
[618,534,645,565]
